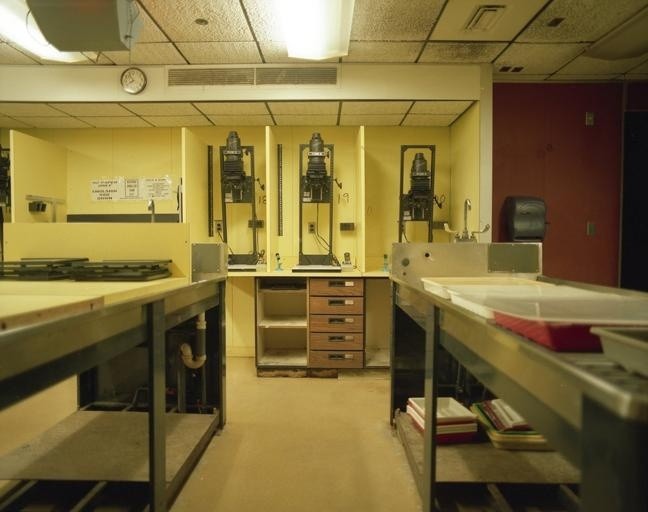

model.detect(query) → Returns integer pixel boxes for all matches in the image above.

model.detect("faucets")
[443,199,490,242]
[147,197,158,223]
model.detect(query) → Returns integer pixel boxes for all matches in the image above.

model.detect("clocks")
[120,67,147,94]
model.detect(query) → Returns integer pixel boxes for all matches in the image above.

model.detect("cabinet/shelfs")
[382,235,646,511]
[255,276,367,371]
[1,239,226,512]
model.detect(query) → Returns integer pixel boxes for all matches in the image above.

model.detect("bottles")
[383,254,388,272]
